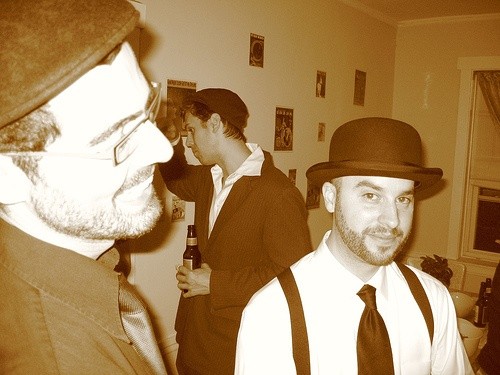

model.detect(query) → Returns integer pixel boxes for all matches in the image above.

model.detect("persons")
[151,86,313,375]
[0,0,176,375]
[234,117,476,375]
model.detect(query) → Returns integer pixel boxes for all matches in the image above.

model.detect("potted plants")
[419,253,452,289]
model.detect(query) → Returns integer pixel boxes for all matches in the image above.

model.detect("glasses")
[0,80,162,168]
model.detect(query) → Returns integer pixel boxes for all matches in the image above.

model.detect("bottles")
[474,277,493,327]
[181,225,202,294]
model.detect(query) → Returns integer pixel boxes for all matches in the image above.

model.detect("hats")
[0,0,141,130]
[305,117,443,196]
[183,88,250,132]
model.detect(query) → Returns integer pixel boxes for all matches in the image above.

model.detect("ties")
[356,283,395,375]
